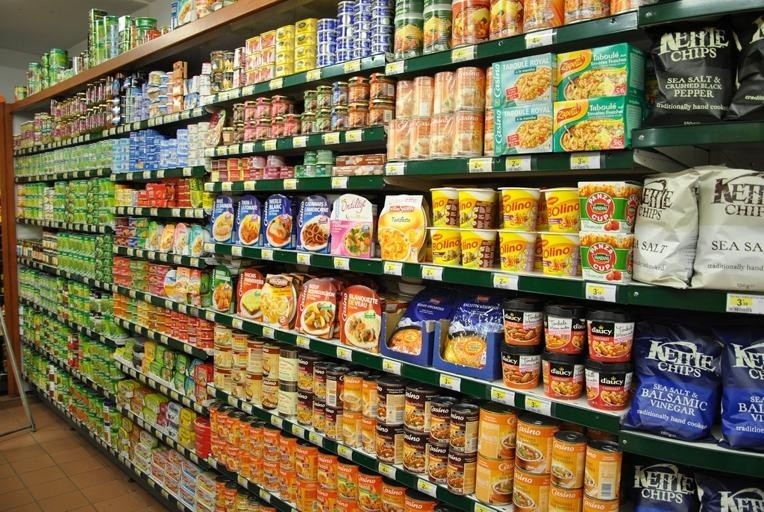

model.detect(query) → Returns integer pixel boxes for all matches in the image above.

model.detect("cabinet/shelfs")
[0,0,763,511]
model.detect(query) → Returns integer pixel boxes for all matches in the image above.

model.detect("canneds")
[210,322,622,512]
[222,72,395,144]
